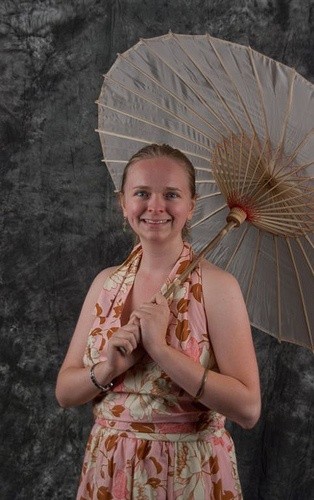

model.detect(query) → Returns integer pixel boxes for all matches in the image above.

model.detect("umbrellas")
[95,30,314,357]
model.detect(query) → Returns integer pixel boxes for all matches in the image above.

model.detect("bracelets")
[90,362,114,392]
[192,368,209,401]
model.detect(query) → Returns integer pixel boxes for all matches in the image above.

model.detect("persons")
[55,144,261,500]
